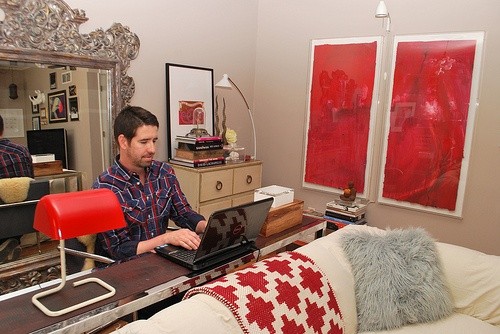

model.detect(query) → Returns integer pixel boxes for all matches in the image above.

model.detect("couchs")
[115,222,500,333]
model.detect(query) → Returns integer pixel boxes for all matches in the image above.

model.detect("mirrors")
[0,49,117,270]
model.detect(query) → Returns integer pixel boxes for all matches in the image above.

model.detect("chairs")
[0,177,48,255]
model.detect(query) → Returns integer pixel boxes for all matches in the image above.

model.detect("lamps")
[377,0,391,32]
[9,69,18,99]
[33,188,129,316]
[214,74,257,160]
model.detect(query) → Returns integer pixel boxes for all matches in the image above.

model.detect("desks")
[0,211,329,334]
[31,169,82,193]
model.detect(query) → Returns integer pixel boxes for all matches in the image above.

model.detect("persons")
[0,114,34,264]
[91,107,208,268]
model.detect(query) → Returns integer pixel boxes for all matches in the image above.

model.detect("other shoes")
[0,238,22,264]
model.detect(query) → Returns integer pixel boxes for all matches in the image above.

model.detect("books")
[169,136,225,168]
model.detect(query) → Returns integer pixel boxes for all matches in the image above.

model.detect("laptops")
[154,197,275,271]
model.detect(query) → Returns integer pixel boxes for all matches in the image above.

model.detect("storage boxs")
[31,154,63,175]
[253,184,304,236]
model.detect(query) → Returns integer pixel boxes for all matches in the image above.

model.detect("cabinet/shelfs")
[160,158,263,225]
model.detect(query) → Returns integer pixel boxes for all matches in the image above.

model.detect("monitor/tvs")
[26,128,68,169]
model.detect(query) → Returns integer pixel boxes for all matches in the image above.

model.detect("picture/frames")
[31,90,68,131]
[166,62,215,161]
